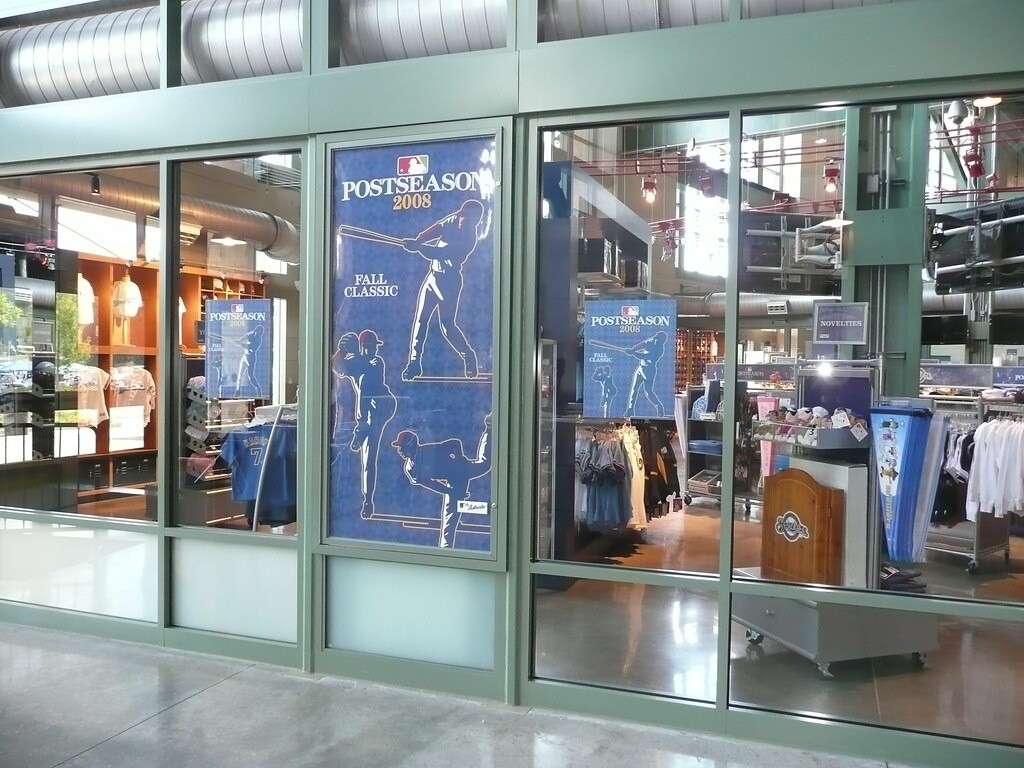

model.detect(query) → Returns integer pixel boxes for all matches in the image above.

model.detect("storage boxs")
[688,469,722,493]
[690,440,721,454]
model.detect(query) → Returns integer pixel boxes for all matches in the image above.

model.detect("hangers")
[576,416,657,440]
[246,403,298,426]
[934,403,1024,430]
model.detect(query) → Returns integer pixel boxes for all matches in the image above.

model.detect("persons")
[113,274,143,318]
[77,272,96,327]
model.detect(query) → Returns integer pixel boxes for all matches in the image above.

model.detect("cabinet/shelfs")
[684,380,765,507]
[0,241,265,496]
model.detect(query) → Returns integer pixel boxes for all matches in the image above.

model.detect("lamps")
[699,176,714,199]
[823,164,842,192]
[85,173,100,196]
[949,100,968,125]
[640,178,656,203]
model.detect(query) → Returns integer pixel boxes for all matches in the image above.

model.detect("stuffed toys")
[753,405,868,447]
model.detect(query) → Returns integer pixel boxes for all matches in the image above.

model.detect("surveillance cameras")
[948,99,968,124]
